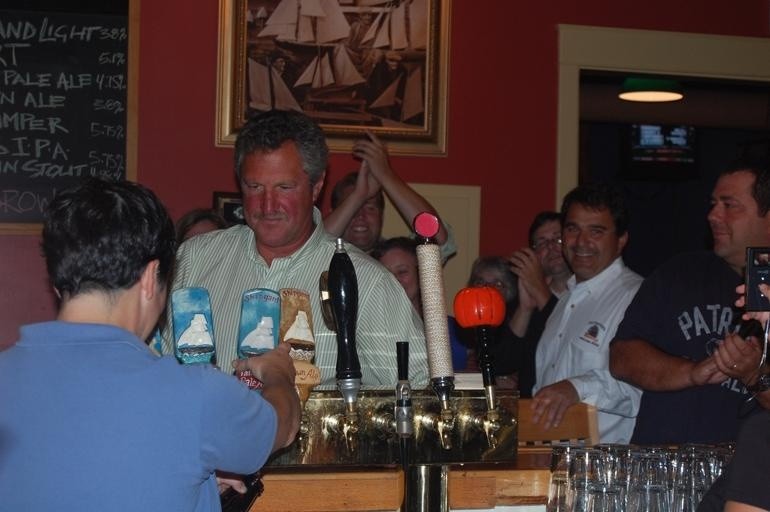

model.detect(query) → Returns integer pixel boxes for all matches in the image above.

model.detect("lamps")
[619,77,684,104]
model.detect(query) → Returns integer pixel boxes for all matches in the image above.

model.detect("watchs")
[747,375,770,394]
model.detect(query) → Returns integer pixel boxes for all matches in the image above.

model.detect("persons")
[0,177,303,511]
[467,183,645,445]
[694,412,770,511]
[734,283,769,324]
[144,108,431,392]
[177,207,228,245]
[372,236,467,373]
[323,129,456,266]
[610,155,769,446]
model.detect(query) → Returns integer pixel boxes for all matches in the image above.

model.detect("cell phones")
[744,247,770,312]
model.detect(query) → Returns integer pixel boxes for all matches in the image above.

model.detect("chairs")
[515,399,598,448]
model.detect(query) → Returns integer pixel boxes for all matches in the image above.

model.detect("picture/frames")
[213,0,451,159]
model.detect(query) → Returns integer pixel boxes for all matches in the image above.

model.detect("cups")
[545,443,735,512]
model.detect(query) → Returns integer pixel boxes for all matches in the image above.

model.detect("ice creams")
[239,316,277,361]
[283,309,316,362]
[177,313,215,364]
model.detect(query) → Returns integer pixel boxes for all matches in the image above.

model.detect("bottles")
[220,475,263,512]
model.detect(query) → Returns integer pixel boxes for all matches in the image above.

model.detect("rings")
[730,364,737,371]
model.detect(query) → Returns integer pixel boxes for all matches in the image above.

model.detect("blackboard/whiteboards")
[0,0,139,237]
